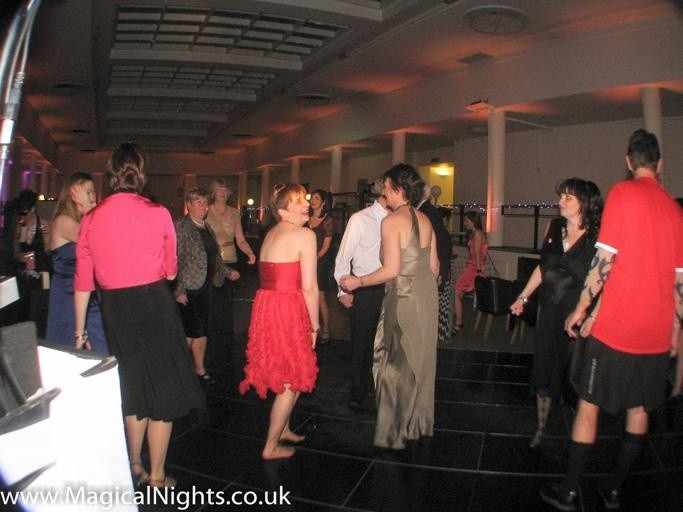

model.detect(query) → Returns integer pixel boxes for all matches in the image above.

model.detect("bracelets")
[517,294,528,304]
[589,315,598,323]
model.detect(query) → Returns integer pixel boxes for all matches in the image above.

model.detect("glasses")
[20,211,29,216]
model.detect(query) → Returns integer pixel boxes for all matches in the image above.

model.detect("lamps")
[468,6,528,35]
[297,94,331,108]
[49,83,81,98]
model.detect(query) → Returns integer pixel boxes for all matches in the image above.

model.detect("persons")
[10,129,490,491]
[540,127,683,512]
[509,178,606,450]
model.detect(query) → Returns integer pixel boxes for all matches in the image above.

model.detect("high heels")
[132,471,150,486]
[198,372,215,385]
[150,476,178,490]
[452,322,464,336]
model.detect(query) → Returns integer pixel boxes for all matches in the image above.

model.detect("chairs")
[469,275,525,346]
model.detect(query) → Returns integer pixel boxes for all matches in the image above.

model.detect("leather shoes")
[598,481,620,507]
[540,482,576,510]
